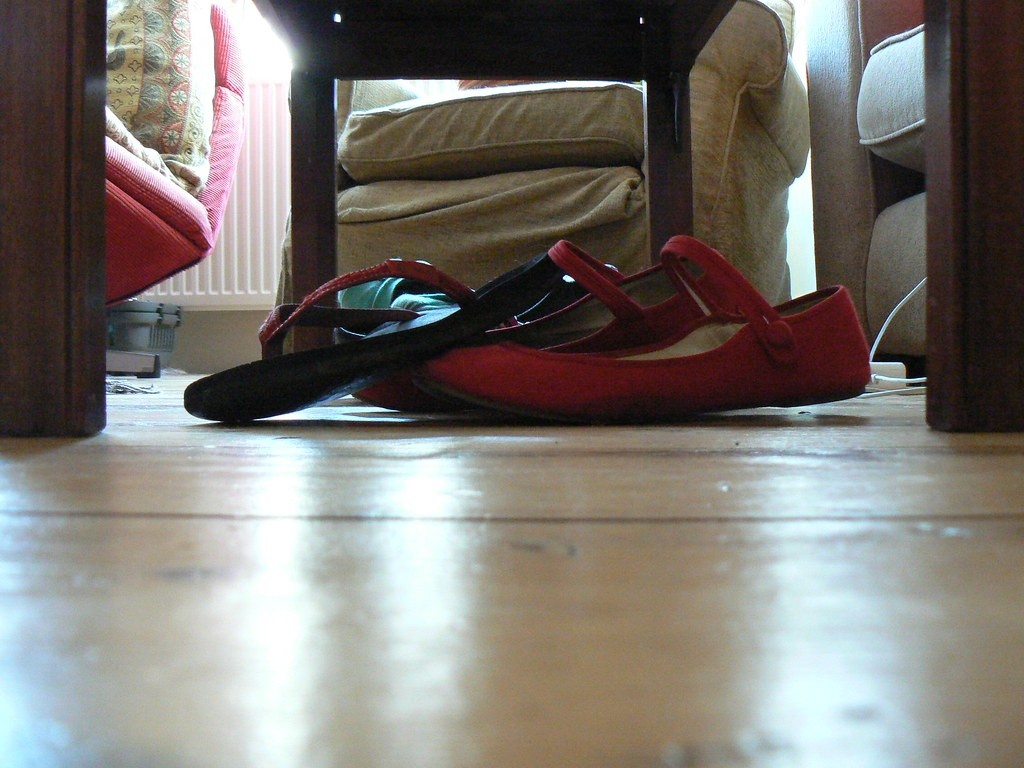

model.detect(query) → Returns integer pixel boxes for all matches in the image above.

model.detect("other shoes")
[354,240,725,414]
[413,234,870,422]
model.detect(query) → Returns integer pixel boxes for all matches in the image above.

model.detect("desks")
[0,0,1024,434]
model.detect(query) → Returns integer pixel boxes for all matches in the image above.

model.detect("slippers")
[183,251,575,420]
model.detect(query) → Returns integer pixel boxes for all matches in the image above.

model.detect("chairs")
[102,0,248,308]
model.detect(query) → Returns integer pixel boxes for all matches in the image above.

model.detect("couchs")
[268,0,810,355]
[861,25,928,385]
